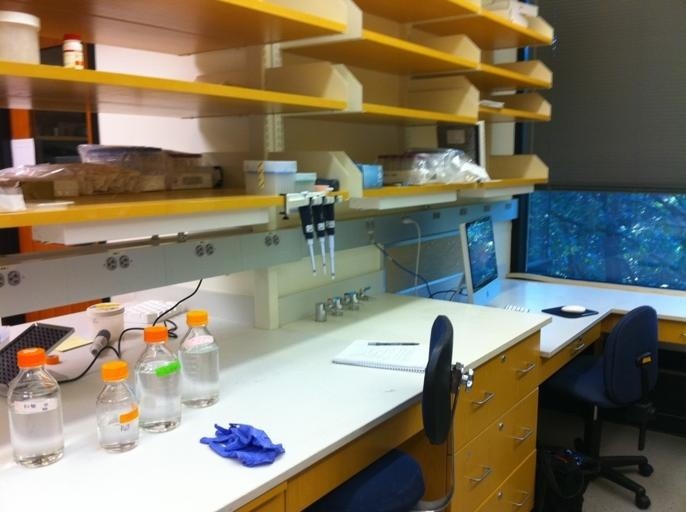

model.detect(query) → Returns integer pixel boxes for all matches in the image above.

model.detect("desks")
[459,278,686,381]
[3,289,553,510]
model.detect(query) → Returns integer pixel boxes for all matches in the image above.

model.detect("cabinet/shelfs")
[1,0,554,230]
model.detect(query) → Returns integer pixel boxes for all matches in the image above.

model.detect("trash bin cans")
[537,445,584,511]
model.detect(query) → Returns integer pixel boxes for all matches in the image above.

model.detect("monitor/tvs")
[459,215,502,305]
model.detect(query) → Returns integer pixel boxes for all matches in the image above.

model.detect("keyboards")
[504,303,529,313]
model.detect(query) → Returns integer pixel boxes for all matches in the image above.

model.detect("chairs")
[305,316,463,508]
[551,306,659,508]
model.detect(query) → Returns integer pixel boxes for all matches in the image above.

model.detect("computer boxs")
[534,448,591,512]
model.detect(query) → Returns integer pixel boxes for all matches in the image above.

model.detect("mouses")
[560,304,586,313]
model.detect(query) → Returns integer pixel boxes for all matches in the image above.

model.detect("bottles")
[96,361,140,455]
[62,32,84,69]
[178,310,221,409]
[86,302,125,343]
[6,347,65,469]
[133,325,181,433]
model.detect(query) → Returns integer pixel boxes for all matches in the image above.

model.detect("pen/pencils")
[370,343,420,346]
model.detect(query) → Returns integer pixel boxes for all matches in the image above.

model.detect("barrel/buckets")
[0,9,42,65]
